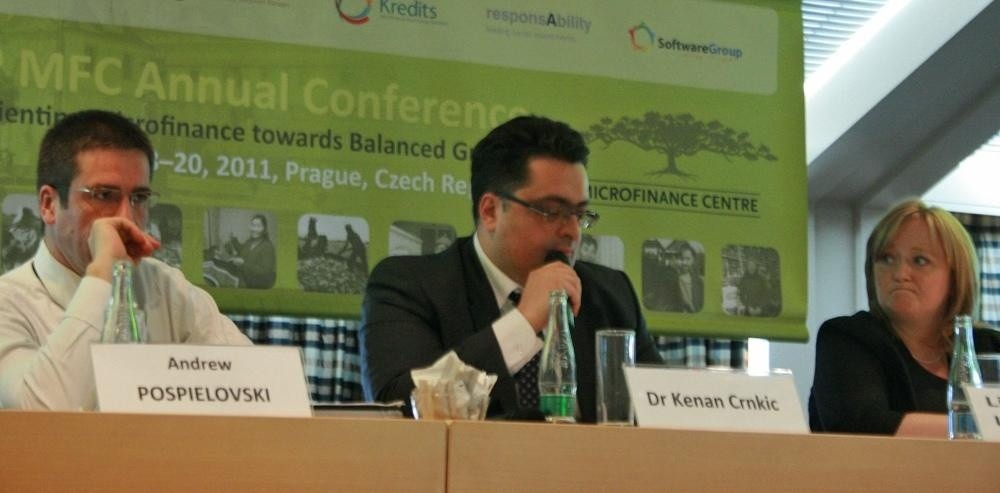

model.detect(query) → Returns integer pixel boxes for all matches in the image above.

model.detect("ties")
[511,294,543,412]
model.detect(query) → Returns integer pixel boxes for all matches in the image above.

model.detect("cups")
[595,330,636,423]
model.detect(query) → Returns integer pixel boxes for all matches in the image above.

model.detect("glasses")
[71,186,164,211]
[491,188,599,231]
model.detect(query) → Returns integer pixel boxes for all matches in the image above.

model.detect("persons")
[640,234,705,313]
[576,232,600,264]
[338,223,369,273]
[0,107,260,415]
[807,199,999,441]
[152,202,182,246]
[220,214,279,288]
[735,256,769,319]
[359,110,670,427]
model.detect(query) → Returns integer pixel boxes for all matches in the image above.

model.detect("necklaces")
[912,350,947,366]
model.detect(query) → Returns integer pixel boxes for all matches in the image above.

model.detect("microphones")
[545,249,575,333]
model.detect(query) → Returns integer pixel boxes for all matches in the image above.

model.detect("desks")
[1,412,451,491]
[449,412,997,488]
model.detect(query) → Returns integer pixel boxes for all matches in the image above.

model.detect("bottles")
[537,290,579,423]
[946,316,983,440]
[102,260,139,343]
